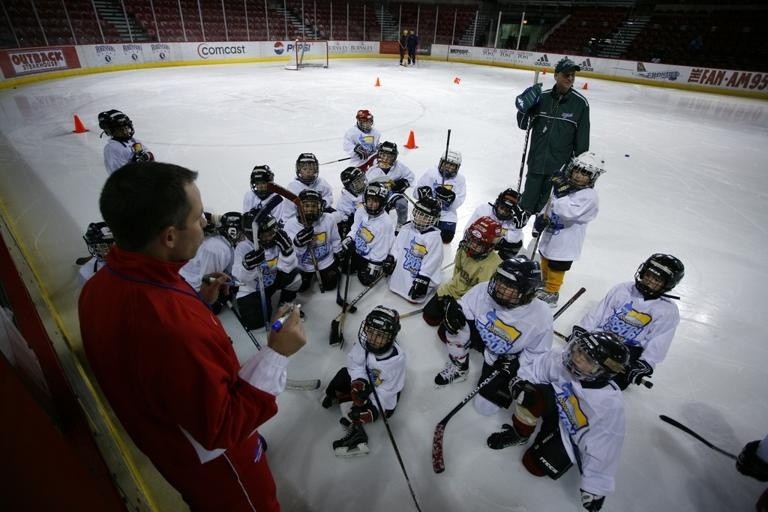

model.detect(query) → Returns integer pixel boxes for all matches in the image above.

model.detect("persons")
[487,331,632,511]
[76,162,308,512]
[530,151,606,307]
[341,109,382,166]
[397,29,409,65]
[98,108,153,175]
[576,253,684,386]
[388,193,451,310]
[420,214,509,344]
[429,255,562,411]
[415,149,466,245]
[463,189,523,262]
[517,58,591,219]
[731,435,766,509]
[179,142,415,330]
[322,305,408,446]
[406,28,418,66]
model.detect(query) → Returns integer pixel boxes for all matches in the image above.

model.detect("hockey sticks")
[253,193,283,347]
[336,272,356,313]
[338,260,351,349]
[432,289,584,472]
[268,181,325,292]
[223,296,319,389]
[329,272,386,345]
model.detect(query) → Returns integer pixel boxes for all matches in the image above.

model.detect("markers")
[203,275,246,287]
[272,303,302,331]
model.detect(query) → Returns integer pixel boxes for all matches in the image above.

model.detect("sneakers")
[333,427,367,451]
[487,424,528,449]
[434,365,469,385]
[278,301,305,317]
[538,293,559,304]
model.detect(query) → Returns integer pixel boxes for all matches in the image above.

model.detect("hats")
[555,59,580,73]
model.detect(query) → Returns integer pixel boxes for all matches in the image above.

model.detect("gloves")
[626,360,654,385]
[580,488,605,512]
[443,295,466,335]
[242,228,314,269]
[408,274,430,299]
[348,380,378,424]
[341,238,355,252]
[550,170,570,197]
[131,151,154,163]
[383,255,395,274]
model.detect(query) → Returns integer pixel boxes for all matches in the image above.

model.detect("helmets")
[562,331,630,381]
[634,253,684,297]
[83,222,115,262]
[98,109,135,141]
[565,152,606,188]
[357,305,401,354]
